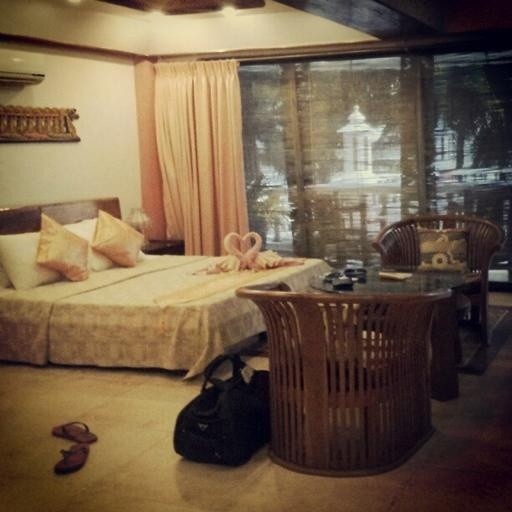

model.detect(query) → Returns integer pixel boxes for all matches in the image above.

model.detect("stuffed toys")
[192,231,305,277]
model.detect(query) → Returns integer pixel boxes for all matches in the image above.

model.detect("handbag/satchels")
[173,354,270,467]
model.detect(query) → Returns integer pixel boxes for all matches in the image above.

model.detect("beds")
[0,197,332,380]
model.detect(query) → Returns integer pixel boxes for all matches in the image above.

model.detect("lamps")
[125,207,149,234]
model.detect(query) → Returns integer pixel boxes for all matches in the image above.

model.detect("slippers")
[51,421,98,474]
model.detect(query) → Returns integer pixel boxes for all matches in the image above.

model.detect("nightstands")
[139,238,186,256]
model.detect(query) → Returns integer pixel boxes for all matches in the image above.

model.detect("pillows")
[0,210,145,291]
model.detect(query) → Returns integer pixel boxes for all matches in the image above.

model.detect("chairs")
[371,214,505,375]
[234,280,453,478]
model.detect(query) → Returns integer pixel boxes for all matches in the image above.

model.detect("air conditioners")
[0,49,45,85]
